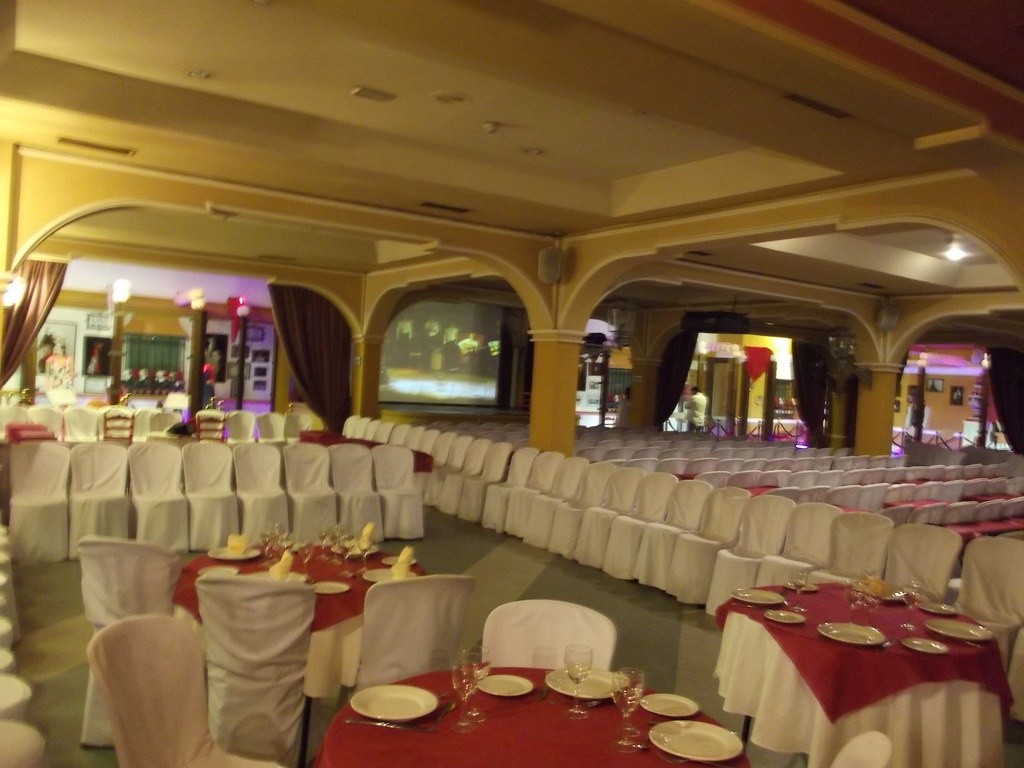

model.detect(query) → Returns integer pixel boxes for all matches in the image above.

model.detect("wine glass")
[792,568,807,594]
[259,521,373,580]
[562,645,592,718]
[449,643,487,734]
[844,571,922,631]
[610,667,645,754]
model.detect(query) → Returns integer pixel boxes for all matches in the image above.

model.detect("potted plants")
[107,379,180,395]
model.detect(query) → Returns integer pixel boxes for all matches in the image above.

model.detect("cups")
[228,533,252,555]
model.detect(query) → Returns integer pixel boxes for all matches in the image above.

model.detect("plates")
[649,720,743,761]
[545,666,631,698]
[381,555,418,567]
[310,581,351,595]
[350,684,439,724]
[762,610,806,624]
[197,565,240,577]
[478,674,533,697]
[362,568,418,584]
[914,601,962,614]
[924,617,994,641]
[816,622,886,646]
[331,543,380,555]
[206,545,260,560]
[901,636,950,655]
[730,588,785,608]
[640,693,699,717]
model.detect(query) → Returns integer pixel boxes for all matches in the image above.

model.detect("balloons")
[174,286,205,311]
[706,342,739,352]
[237,306,250,316]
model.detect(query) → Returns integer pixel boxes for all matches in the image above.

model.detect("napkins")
[271,549,294,581]
[227,534,252,554]
[360,520,373,550]
[390,546,412,580]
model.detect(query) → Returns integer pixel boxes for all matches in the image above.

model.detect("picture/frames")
[948,384,964,407]
[227,325,271,391]
[925,377,945,393]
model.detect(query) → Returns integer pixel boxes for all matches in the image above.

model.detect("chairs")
[0,392,618,768]
[342,414,1024,722]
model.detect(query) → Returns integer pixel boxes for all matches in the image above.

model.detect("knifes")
[346,718,432,733]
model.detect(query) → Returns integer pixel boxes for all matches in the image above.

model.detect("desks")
[174,540,424,698]
[143,431,199,443]
[4,424,58,444]
[713,583,1015,768]
[299,430,434,472]
[311,667,751,768]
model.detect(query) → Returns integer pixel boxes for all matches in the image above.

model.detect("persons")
[616,386,631,424]
[685,386,708,433]
[953,388,962,403]
[397,312,488,356]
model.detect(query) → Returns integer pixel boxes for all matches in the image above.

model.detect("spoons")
[434,700,457,725]
